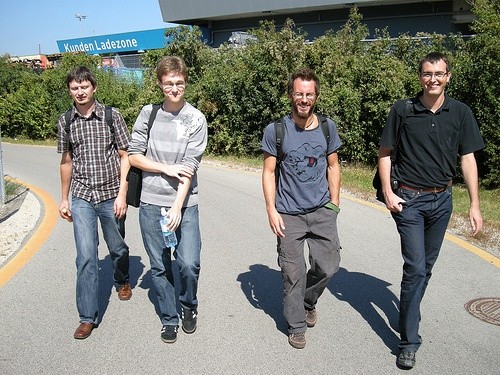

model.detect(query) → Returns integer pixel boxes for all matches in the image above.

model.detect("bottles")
[159,206,178,248]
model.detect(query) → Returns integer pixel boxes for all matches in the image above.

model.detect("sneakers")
[181,306,198,333]
[304,307,317,325]
[161,325,179,342]
[288,333,306,348]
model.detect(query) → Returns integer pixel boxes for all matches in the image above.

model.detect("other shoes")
[419,307,421,322]
[397,349,416,368]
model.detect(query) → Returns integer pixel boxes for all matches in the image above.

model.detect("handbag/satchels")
[372,161,394,204]
[126,166,142,208]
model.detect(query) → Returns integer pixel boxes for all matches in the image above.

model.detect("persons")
[128,55,208,343]
[57,64,132,338]
[260,68,343,349]
[378,52,484,370]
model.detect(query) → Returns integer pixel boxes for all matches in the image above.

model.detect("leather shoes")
[118,284,132,301]
[74,322,95,339]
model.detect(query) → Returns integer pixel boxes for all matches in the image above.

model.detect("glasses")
[419,71,448,78]
[290,92,316,98]
[160,80,185,88]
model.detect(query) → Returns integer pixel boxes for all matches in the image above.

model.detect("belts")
[400,180,452,193]
